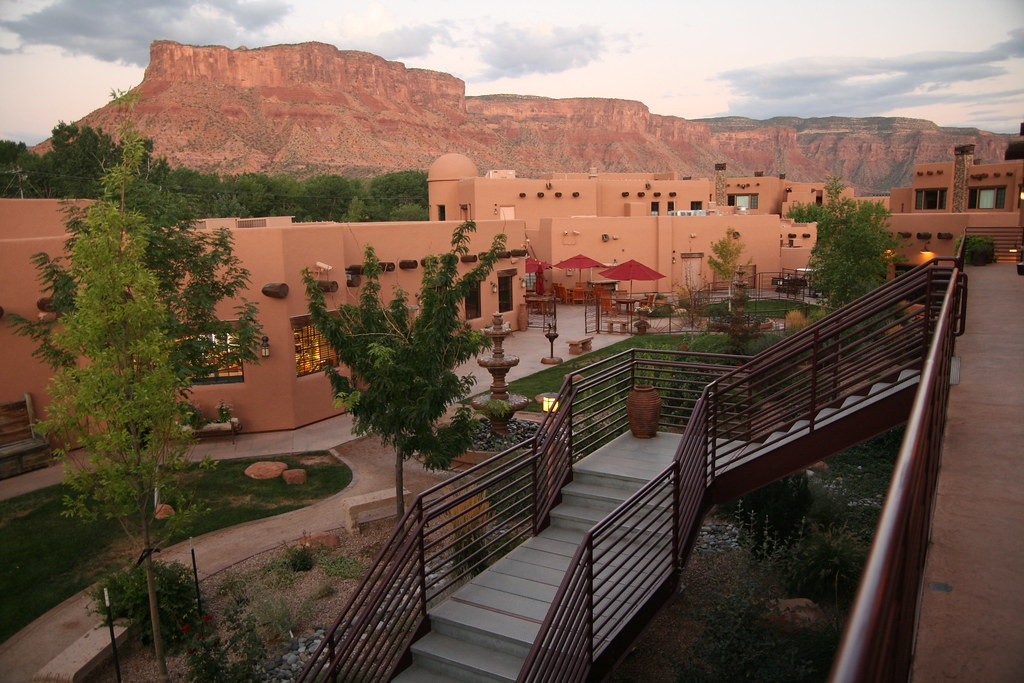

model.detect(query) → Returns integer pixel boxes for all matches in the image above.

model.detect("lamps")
[494,208,498,215]
[566,335,594,354]
[490,281,497,293]
[260,336,270,357]
[519,278,526,288]
[672,257,676,263]
[543,389,560,412]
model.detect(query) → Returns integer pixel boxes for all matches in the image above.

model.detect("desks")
[618,296,641,315]
[526,296,560,316]
[569,288,593,305]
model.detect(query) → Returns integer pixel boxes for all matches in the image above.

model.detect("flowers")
[184,399,203,429]
[634,321,651,329]
[635,306,653,314]
[216,400,233,422]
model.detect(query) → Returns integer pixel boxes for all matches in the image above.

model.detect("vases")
[637,326,646,334]
[638,311,647,316]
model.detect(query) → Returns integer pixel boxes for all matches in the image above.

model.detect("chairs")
[571,287,585,305]
[771,277,807,294]
[601,289,617,319]
[559,286,572,304]
[587,283,601,305]
[640,294,655,309]
[552,282,560,297]
[576,281,586,288]
[615,289,629,312]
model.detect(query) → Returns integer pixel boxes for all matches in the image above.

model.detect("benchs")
[175,417,242,448]
[0,392,55,480]
[31,618,134,683]
[341,486,412,537]
[604,319,628,333]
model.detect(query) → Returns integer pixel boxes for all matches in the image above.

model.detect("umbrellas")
[525,258,552,289]
[553,254,607,288]
[535,265,545,298]
[599,259,667,299]
